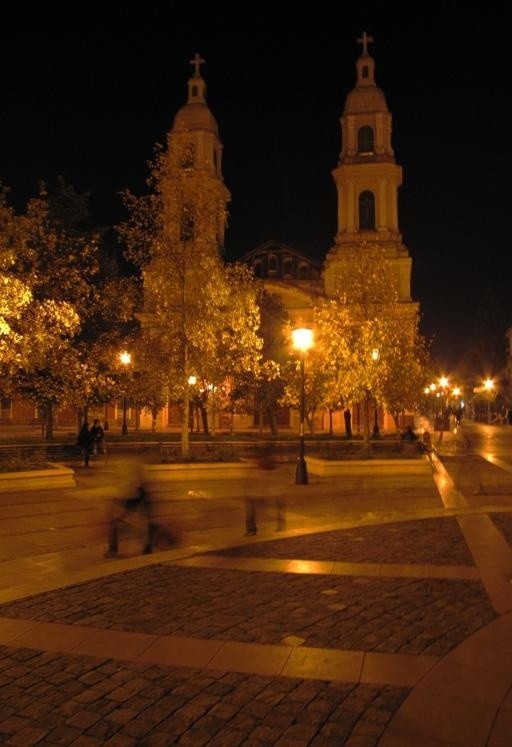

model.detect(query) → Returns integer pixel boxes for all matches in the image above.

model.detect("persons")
[100,458,164,556]
[449,415,490,500]
[401,425,417,441]
[238,437,287,535]
[76,420,104,467]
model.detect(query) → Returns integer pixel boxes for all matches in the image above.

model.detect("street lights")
[412,373,496,428]
[121,351,130,434]
[370,346,381,439]
[188,375,197,431]
[288,325,317,485]
